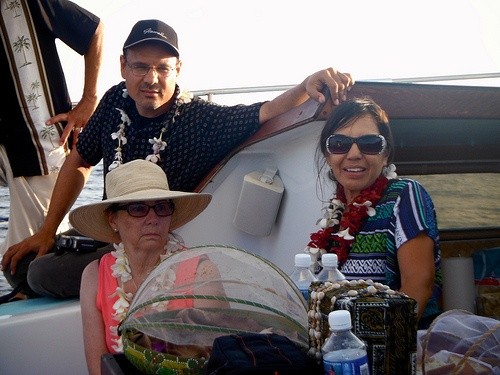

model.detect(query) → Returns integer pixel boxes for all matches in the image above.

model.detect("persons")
[0,0,104,254]
[0,18,355,305]
[77,156,253,375]
[288,98,442,331]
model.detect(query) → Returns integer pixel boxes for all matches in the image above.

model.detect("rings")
[74,127,82,131]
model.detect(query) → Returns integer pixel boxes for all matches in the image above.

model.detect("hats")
[123,19,179,56]
[69,159,211,244]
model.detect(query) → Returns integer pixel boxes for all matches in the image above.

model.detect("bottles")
[320,310,370,375]
[317,253,346,282]
[287,254,319,303]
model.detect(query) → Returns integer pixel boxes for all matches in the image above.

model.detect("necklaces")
[129,259,162,294]
[110,235,182,354]
[303,159,404,282]
[109,74,192,176]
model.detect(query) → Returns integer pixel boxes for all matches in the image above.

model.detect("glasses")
[116,201,177,218]
[326,133,387,155]
[122,56,179,78]
